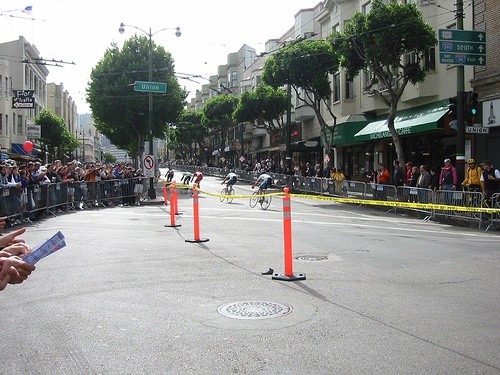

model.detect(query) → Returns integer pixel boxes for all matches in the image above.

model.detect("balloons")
[23,140,33,152]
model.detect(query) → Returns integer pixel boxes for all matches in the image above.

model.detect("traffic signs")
[438,29,487,65]
[11,97,35,109]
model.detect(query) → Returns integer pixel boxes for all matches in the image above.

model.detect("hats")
[444,159,451,162]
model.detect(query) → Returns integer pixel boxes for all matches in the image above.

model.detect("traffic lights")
[449,96,456,118]
[293,130,298,136]
[467,93,478,116]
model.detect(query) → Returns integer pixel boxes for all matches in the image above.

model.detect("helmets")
[468,158,475,164]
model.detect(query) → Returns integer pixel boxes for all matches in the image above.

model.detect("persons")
[181,173,191,184]
[165,166,174,182]
[252,171,272,202]
[0,159,146,225]
[0,218,35,291]
[220,157,233,171]
[191,171,203,188]
[240,158,347,195]
[368,159,500,220]
[221,171,237,193]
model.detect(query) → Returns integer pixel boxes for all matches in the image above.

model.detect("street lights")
[119,23,181,154]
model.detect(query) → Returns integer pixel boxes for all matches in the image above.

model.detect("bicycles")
[249,185,272,210]
[219,182,235,203]
[165,175,200,196]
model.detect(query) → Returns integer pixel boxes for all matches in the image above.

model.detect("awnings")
[212,147,229,155]
[320,122,369,146]
[13,144,37,157]
[354,103,452,142]
